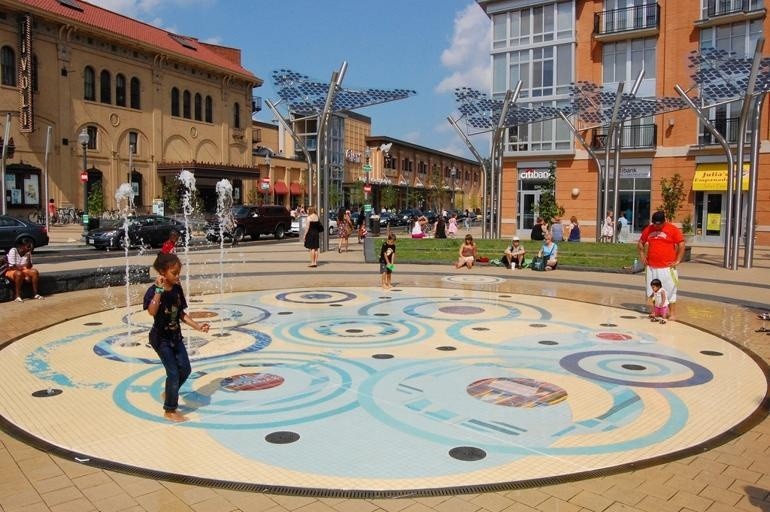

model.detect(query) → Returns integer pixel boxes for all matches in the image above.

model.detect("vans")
[204,204,292,243]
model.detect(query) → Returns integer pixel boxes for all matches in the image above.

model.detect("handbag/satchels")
[316,214,325,233]
[531,255,550,272]
[0,254,10,277]
[500,246,513,268]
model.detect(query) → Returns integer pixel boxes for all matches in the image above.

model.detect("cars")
[87,215,192,251]
[0,215,50,254]
[286,207,498,237]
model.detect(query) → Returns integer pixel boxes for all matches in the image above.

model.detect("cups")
[511,261,516,271]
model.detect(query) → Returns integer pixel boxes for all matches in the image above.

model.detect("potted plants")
[743,224,758,248]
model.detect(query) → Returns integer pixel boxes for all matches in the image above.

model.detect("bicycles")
[29,207,82,225]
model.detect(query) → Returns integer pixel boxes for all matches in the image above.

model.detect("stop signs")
[79,171,89,183]
[363,184,372,192]
[263,176,270,184]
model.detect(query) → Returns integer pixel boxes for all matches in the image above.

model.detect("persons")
[142,254,211,423]
[503,237,527,270]
[618,210,628,244]
[646,278,669,325]
[537,232,558,270]
[288,204,497,254]
[601,210,615,243]
[548,216,564,241]
[566,216,581,242]
[532,216,548,240]
[379,233,398,288]
[161,230,180,255]
[456,234,480,269]
[637,211,687,322]
[2,238,46,303]
[302,206,321,268]
[48,198,58,227]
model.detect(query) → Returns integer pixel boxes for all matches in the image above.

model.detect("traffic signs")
[363,165,372,171]
[362,203,372,212]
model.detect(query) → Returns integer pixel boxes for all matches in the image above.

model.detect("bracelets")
[150,299,161,305]
[154,286,164,294]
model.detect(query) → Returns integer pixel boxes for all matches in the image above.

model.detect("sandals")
[31,294,44,300]
[14,297,24,304]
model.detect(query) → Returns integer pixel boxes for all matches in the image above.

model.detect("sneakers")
[163,408,187,423]
[307,263,318,267]
[160,392,166,402]
[651,317,668,325]
[338,240,362,254]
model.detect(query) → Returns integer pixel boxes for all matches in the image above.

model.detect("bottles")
[633,258,638,269]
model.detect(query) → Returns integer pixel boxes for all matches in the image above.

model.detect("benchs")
[0,265,149,302]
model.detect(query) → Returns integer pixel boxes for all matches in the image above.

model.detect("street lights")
[262,152,272,205]
[80,126,90,222]
[362,145,372,233]
[450,166,457,210]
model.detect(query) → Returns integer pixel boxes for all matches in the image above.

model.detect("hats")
[512,236,520,241]
[652,211,666,224]
